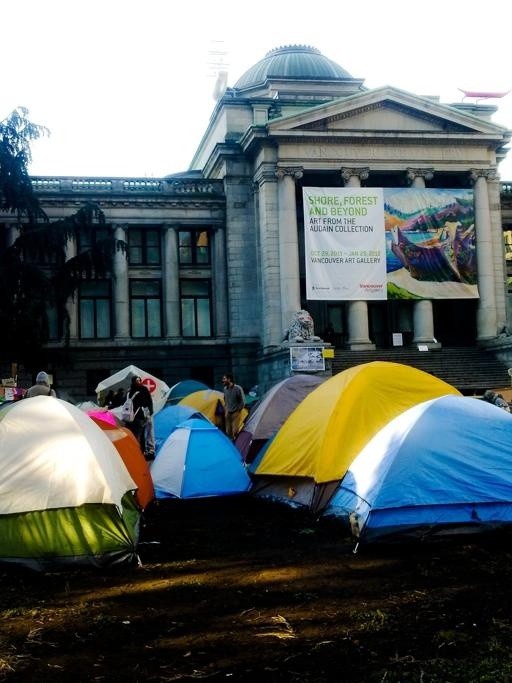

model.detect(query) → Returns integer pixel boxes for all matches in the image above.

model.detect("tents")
[0,364,512,575]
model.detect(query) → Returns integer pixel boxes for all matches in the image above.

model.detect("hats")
[36,372,48,381]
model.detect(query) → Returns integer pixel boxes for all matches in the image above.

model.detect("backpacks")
[121,390,142,422]
